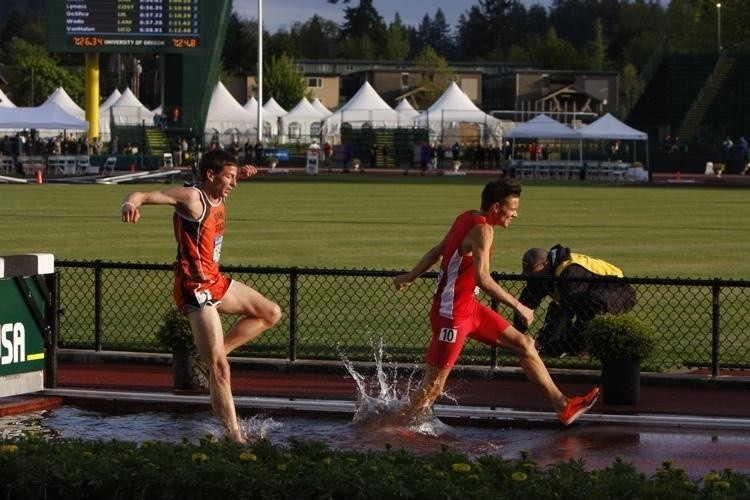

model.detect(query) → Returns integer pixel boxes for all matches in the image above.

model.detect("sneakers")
[556,386,601,427]
[185,352,209,389]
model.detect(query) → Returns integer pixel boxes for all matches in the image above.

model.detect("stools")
[159,153,173,167]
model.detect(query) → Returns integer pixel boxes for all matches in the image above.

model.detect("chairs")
[0,153,116,177]
[513,160,633,181]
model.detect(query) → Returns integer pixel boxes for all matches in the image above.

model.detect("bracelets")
[121,202,135,208]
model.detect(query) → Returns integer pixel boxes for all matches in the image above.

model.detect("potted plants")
[152,308,211,390]
[589,310,659,404]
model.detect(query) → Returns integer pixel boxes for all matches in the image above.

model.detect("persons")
[452,140,550,179]
[368,141,446,171]
[153,112,168,130]
[121,150,282,445]
[511,243,637,356]
[323,142,333,166]
[722,136,748,156]
[2,132,139,155]
[172,137,263,168]
[393,179,600,439]
[608,140,623,163]
[309,141,320,152]
[663,133,680,154]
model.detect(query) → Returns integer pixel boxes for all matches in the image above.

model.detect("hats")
[521,248,548,275]
